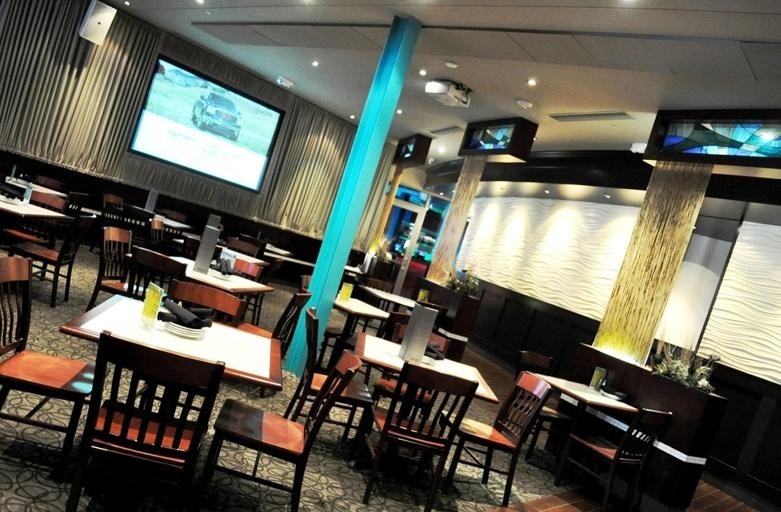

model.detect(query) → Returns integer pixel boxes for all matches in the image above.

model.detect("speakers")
[78,0,117,46]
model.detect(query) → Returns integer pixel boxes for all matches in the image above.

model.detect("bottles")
[24,185,33,205]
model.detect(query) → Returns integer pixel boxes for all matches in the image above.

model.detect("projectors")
[424,79,472,108]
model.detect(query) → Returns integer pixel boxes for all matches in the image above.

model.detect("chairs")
[366,323,450,420]
[360,359,478,512]
[436,368,554,512]
[265,243,469,387]
[282,308,373,458]
[567,399,681,512]
[66,329,225,512]
[0,257,113,467]
[202,348,367,512]
[515,351,572,463]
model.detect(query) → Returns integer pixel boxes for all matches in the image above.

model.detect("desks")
[59,294,284,393]
[528,372,637,488]
[352,330,499,488]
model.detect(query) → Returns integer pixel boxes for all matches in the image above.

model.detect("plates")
[0,196,19,205]
[601,389,622,401]
[165,322,205,340]
[422,356,437,363]
[209,268,231,281]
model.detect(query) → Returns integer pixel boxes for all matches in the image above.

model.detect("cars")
[191,92,243,142]
[391,227,432,264]
